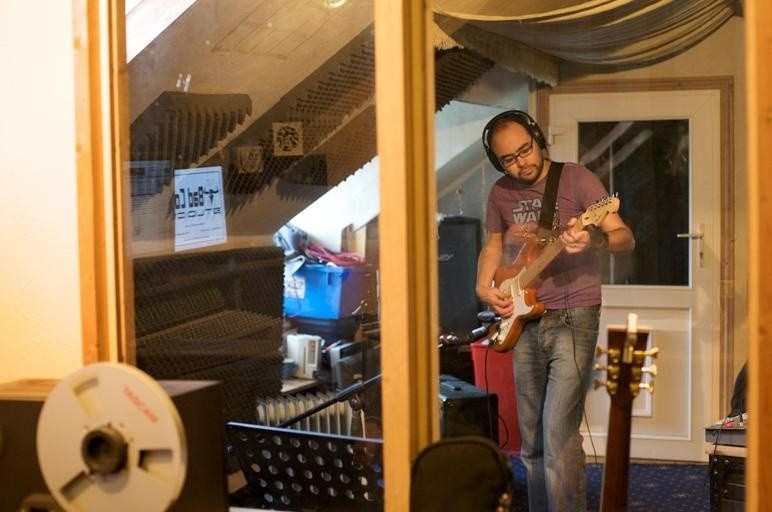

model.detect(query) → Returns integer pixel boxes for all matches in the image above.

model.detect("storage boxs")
[283,257,373,321]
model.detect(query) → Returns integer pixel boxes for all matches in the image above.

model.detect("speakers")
[440,374,500,449]
[438,217,484,345]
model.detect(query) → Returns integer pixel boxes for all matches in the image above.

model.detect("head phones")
[482,109,547,173]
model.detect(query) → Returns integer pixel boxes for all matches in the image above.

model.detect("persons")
[476,109,636,511]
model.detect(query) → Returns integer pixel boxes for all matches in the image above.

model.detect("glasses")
[497,135,533,169]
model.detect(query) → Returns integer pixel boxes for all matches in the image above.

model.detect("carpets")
[506,453,713,512]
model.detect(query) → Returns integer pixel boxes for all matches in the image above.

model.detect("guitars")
[593,312,660,511]
[486,192,620,352]
